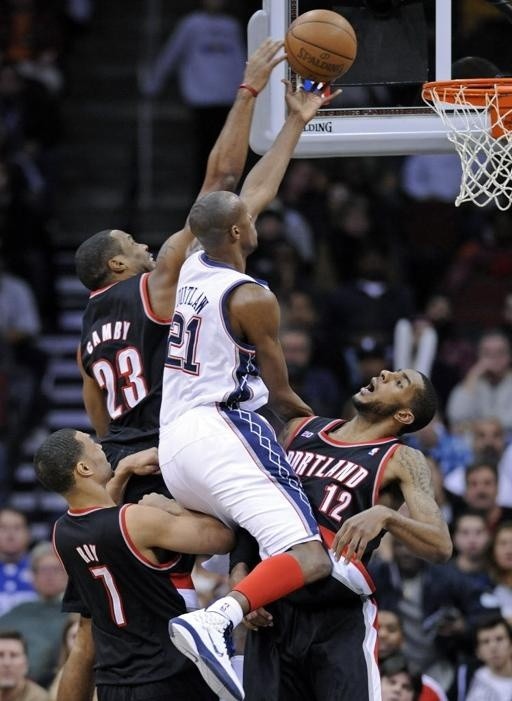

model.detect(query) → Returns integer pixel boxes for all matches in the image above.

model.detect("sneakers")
[168,607,248,701]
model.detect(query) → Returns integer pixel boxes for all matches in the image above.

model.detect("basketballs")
[284,9,358,84]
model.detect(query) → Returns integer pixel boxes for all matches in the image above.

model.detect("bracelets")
[239,83,259,97]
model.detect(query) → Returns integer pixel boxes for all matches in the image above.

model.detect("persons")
[294,159,328,368]
[230,367,453,701]
[331,155,454,369]
[35,428,234,701]
[157,67,333,701]
[55,41,292,701]
[370,540,452,701]
[157,1,243,201]
[0,508,44,620]
[0,631,50,701]
[0,542,82,691]
[249,201,291,287]
[454,208,510,701]
[2,267,50,502]
